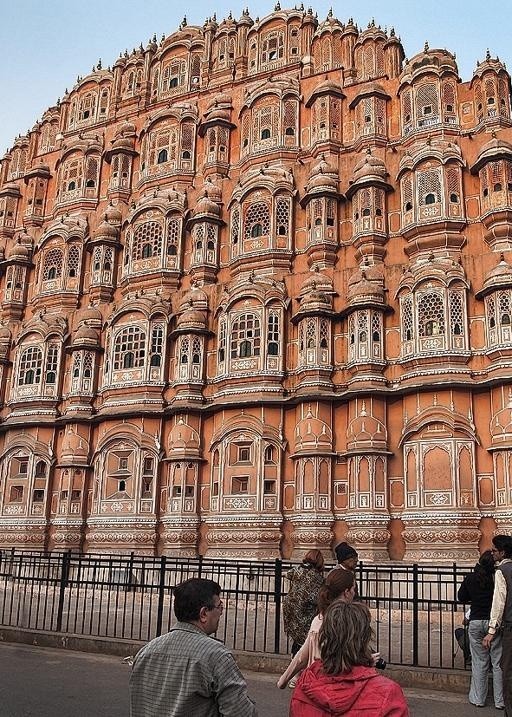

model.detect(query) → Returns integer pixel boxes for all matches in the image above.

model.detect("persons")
[280,547,329,686]
[275,568,381,689]
[456,548,505,708]
[329,541,363,600]
[455,608,474,666]
[127,577,259,717]
[287,599,410,717]
[481,534,512,716]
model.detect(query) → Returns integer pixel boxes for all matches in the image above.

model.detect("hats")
[335,542,358,564]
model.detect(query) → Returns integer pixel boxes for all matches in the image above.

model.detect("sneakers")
[494,703,505,710]
[468,698,485,707]
